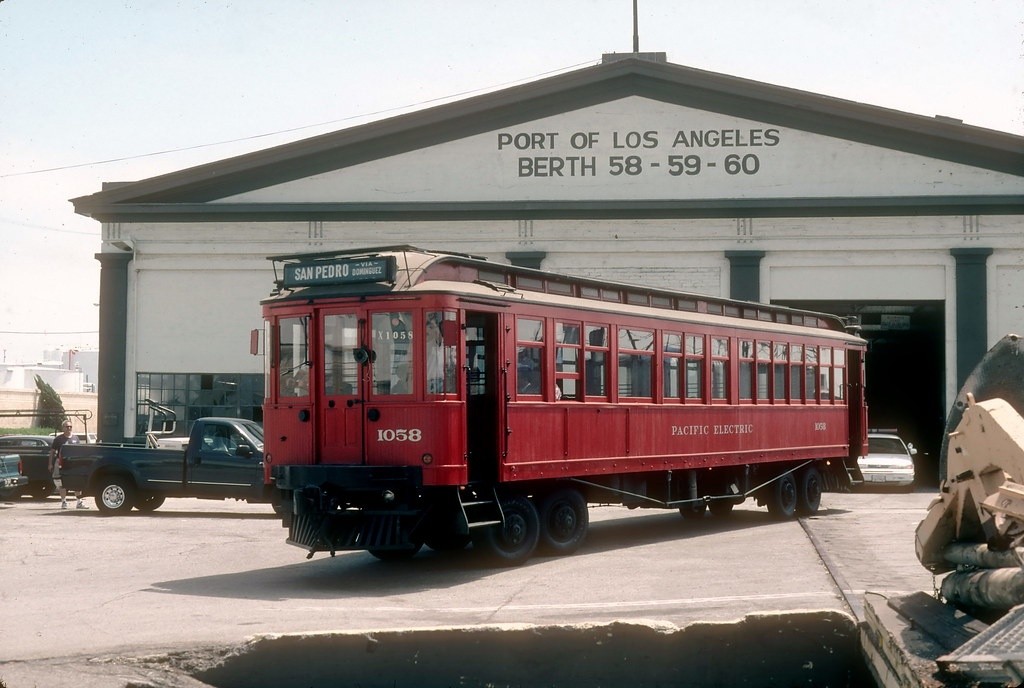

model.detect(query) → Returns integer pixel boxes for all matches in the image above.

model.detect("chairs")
[213,433,224,446]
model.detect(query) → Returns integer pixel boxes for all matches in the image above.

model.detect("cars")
[0,435,60,502]
[856,429,918,492]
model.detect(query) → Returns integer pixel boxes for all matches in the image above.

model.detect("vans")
[44,431,98,445]
[157,437,217,452]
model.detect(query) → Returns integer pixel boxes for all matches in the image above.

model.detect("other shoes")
[61,501,68,509]
[76,502,89,509]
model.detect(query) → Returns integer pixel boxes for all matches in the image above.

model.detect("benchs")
[158,437,212,450]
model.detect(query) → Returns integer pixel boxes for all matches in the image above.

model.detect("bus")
[250,244,870,569]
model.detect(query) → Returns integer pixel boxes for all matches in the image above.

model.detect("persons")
[48,420,91,510]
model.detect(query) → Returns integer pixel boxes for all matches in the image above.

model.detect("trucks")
[57,417,285,518]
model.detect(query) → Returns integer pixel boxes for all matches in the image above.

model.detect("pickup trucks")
[0,453,30,500]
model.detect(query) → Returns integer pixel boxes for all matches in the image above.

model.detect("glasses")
[63,426,72,429]
